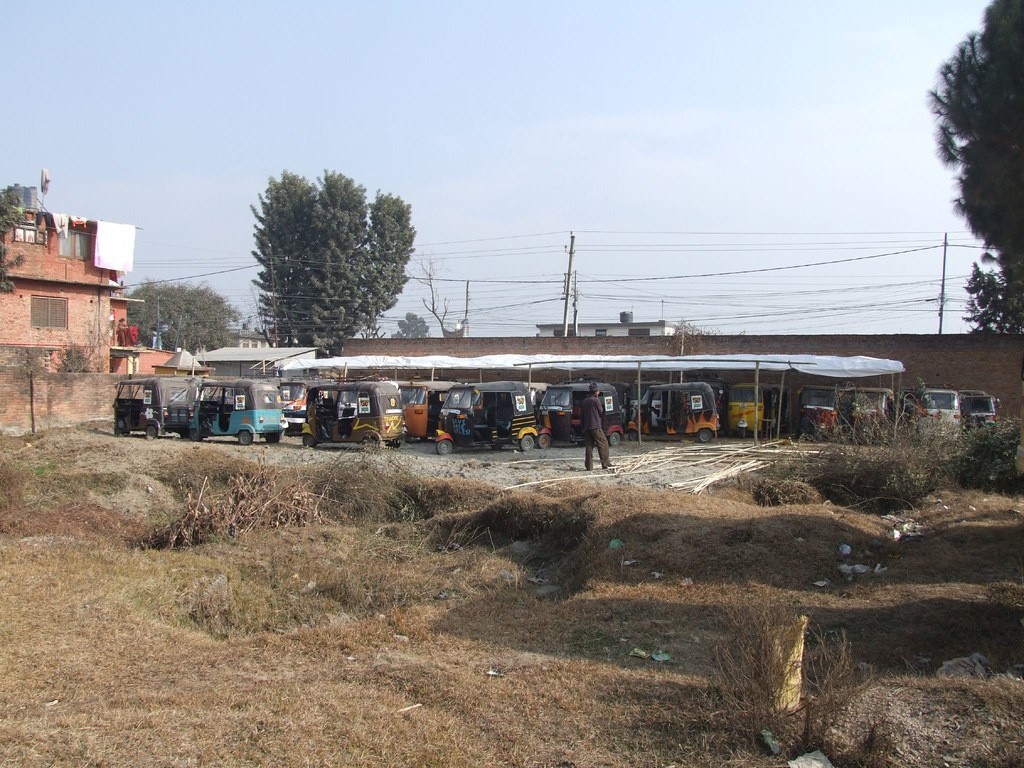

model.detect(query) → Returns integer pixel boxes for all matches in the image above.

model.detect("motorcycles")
[797,384,842,442]
[187,377,289,446]
[625,381,721,443]
[725,382,789,439]
[844,386,898,438]
[536,375,632,451]
[958,389,1002,432]
[112,377,194,441]
[275,376,339,436]
[913,388,963,439]
[397,379,463,441]
[300,379,408,452]
[435,380,542,456]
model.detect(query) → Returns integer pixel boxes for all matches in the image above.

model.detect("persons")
[582,382,616,471]
[116,318,129,347]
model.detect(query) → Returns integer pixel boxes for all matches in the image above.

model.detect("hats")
[589,381,600,391]
